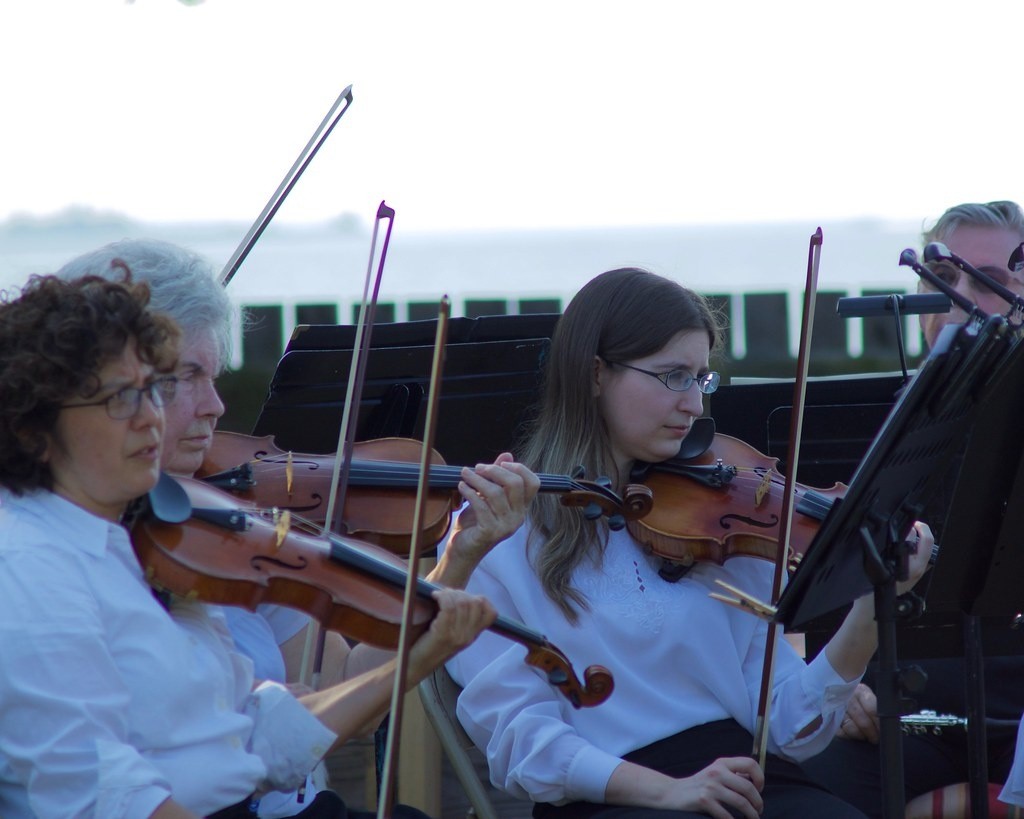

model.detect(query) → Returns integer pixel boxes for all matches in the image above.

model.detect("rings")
[842,718,851,725]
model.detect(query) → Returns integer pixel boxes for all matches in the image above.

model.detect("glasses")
[921,264,1023,293]
[601,357,720,394]
[60,376,177,421]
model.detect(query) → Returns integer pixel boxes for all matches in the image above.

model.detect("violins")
[135,470,615,710]
[626,433,944,568]
[195,430,655,557]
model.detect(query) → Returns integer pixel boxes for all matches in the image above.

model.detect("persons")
[0,238,541,819]
[437,268,935,819]
[805,201,1024,819]
